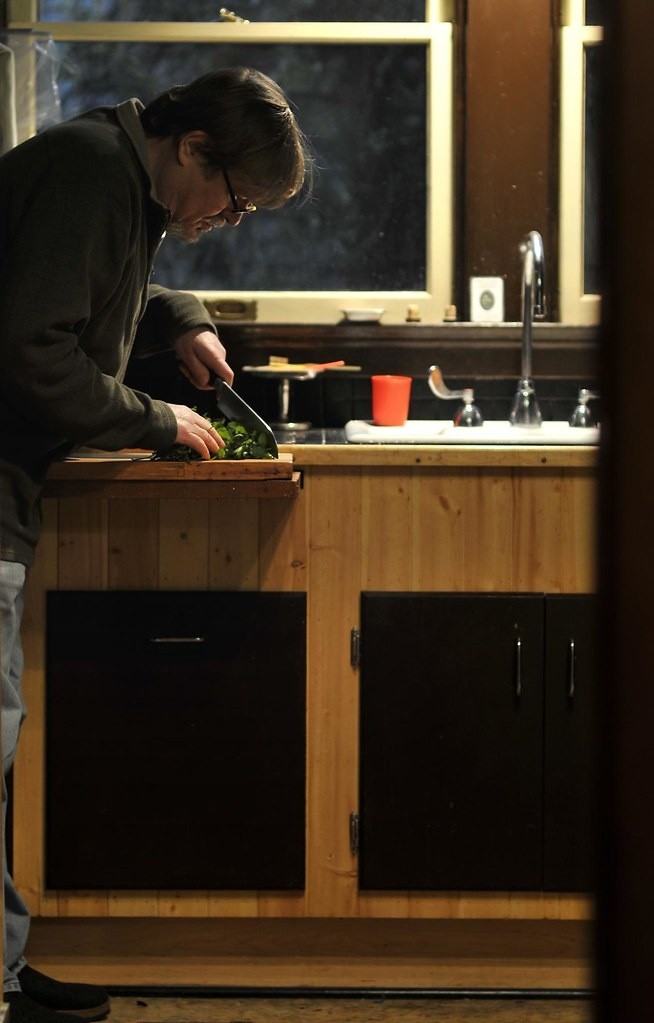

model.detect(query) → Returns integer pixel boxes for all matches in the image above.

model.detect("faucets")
[511,230,546,429]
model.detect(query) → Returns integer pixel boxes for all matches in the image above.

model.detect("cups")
[370,375,413,425]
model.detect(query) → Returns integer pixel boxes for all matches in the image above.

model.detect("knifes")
[207,368,279,458]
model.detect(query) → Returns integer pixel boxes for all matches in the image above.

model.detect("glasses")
[223,168,256,215]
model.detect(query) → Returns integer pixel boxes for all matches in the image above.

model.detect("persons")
[0,66,310,1023]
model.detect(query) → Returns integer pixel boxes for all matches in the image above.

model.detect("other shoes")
[2,965,111,1023]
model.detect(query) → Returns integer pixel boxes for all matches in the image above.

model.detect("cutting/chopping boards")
[63,449,293,480]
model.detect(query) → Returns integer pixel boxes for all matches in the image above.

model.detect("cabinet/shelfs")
[13,428,609,996]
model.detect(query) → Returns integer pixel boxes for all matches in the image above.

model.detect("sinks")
[344,416,599,444]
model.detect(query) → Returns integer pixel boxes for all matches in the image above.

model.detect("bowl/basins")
[340,306,386,323]
